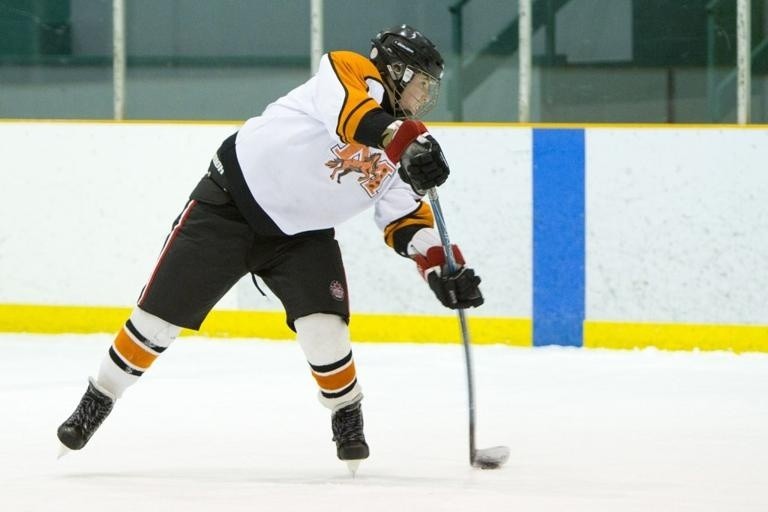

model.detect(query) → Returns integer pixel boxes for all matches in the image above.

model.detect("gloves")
[424,262,484,310]
[398,130,449,190]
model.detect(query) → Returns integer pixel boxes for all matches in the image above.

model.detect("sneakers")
[57,377,115,450]
[332,394,369,459]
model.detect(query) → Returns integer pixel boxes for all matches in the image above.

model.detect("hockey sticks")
[409,149,512,471]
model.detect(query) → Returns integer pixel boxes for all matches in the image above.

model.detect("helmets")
[370,24,445,120]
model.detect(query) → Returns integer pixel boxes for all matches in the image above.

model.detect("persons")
[56,22,484,460]
